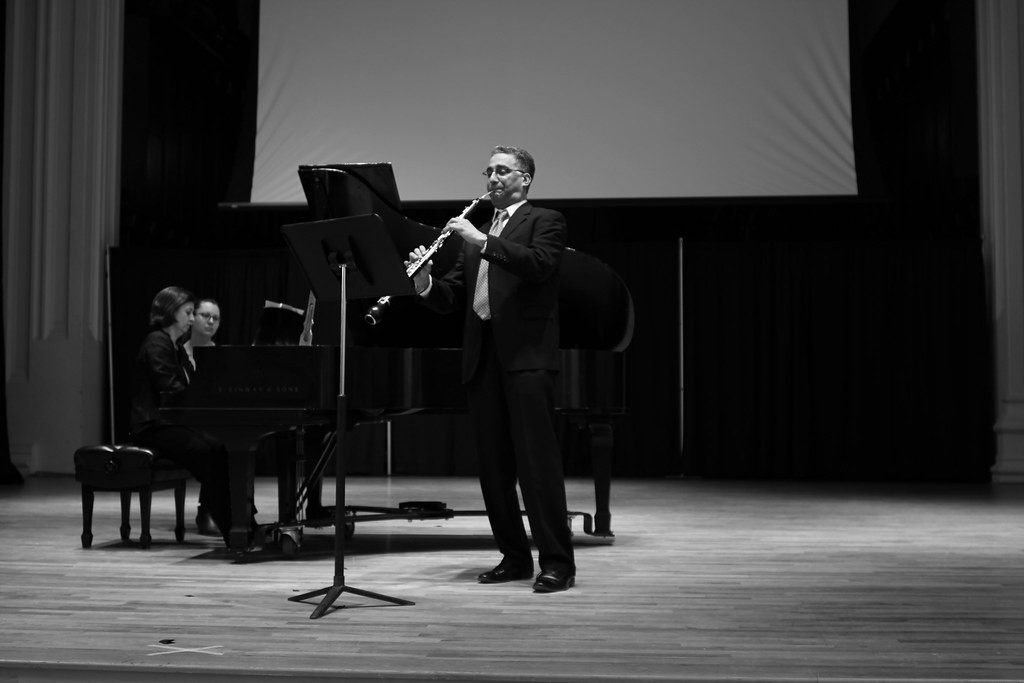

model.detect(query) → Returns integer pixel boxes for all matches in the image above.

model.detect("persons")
[403,148,579,592]
[183,300,225,538]
[124,286,263,560]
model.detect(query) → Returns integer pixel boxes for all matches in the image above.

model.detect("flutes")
[363,189,496,327]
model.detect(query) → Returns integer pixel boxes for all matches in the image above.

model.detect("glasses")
[196,311,220,321]
[482,168,525,176]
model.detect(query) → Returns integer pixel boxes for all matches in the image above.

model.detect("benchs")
[73,441,195,551]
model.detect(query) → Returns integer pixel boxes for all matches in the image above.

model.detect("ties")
[473,210,508,320]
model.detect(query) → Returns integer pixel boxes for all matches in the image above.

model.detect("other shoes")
[258,523,274,536]
[196,505,221,536]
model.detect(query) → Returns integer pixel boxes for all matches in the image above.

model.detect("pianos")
[157,162,638,559]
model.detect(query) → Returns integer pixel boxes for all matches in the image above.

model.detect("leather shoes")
[477,561,534,583]
[532,568,575,593]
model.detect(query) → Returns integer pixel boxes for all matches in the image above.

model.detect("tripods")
[281,212,416,617]
